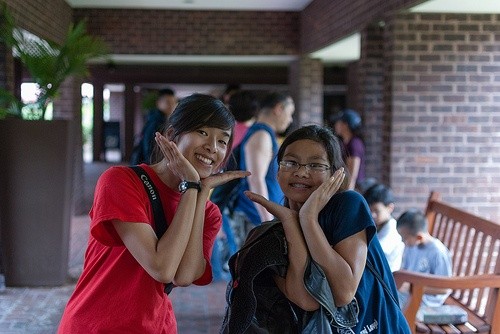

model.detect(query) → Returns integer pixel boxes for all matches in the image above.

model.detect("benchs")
[393,191,500,334]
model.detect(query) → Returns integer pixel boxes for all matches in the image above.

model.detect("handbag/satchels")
[126,146,143,165]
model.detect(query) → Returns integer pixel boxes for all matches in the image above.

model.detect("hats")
[329,109,360,127]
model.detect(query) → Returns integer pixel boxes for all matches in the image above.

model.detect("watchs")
[178,179,202,194]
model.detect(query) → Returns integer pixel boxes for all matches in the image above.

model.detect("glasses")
[279,160,332,171]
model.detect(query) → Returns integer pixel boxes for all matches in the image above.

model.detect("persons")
[132,89,177,166]
[334,110,365,192]
[245,123,412,334]
[58,93,252,334]
[206,93,259,282]
[234,92,296,226]
[395,207,454,322]
[363,183,406,273]
